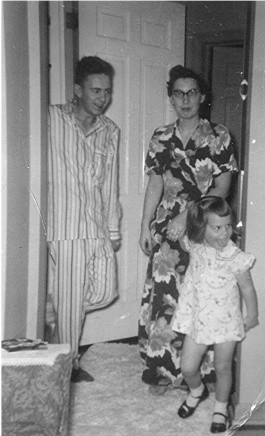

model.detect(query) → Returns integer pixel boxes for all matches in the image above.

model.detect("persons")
[135,65,239,396]
[41,55,123,383]
[167,196,259,434]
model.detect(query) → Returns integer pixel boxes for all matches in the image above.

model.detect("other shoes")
[178,386,209,418]
[211,411,228,433]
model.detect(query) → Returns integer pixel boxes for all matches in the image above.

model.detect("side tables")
[1,339,73,436]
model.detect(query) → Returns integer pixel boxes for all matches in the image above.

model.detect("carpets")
[71,343,232,435]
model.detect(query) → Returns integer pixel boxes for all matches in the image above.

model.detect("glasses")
[171,88,201,96]
